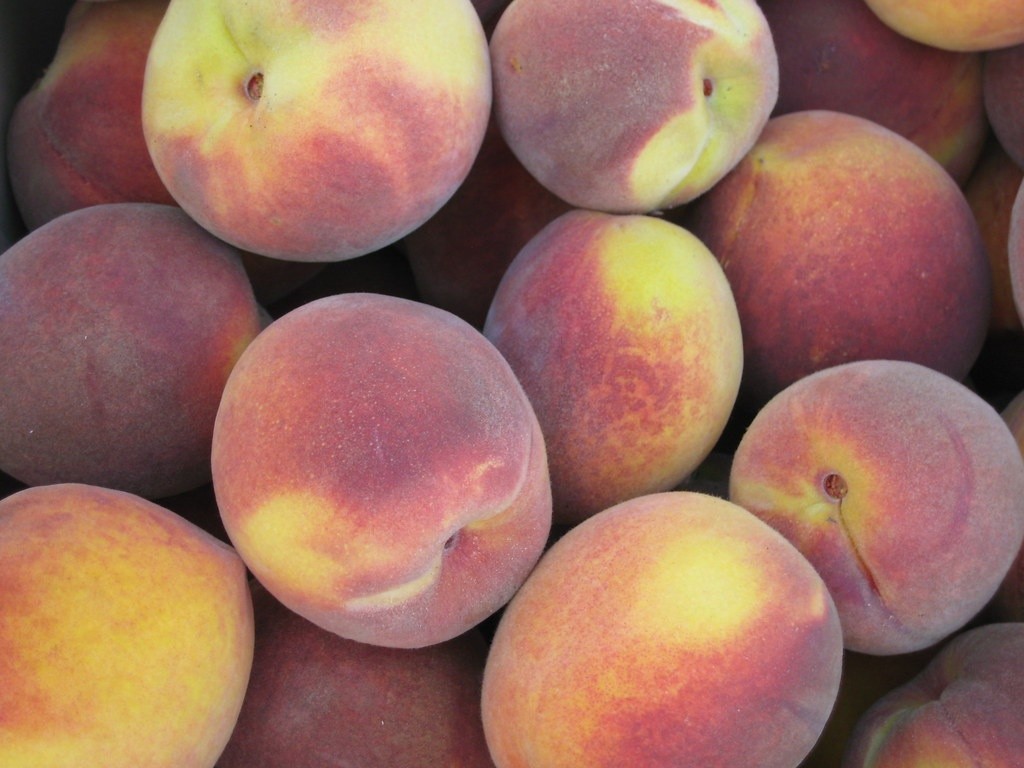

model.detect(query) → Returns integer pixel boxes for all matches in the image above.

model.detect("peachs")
[0,0,1024,768]
